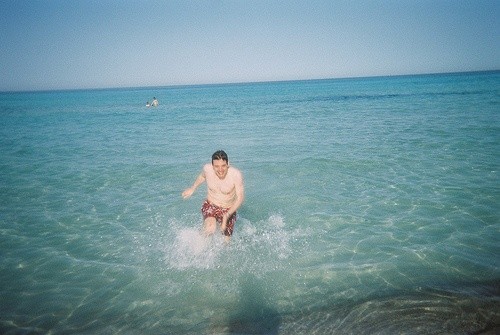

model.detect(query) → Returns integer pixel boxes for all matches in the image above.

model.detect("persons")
[146,102,150,107]
[151,97,158,106]
[181,150,244,244]
